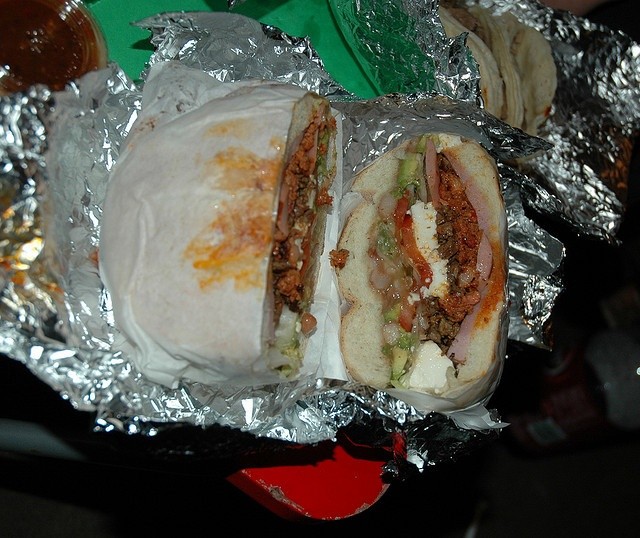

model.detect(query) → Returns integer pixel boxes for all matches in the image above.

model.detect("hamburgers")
[435,4,559,138]
[97,89,336,386]
[328,132,508,394]
